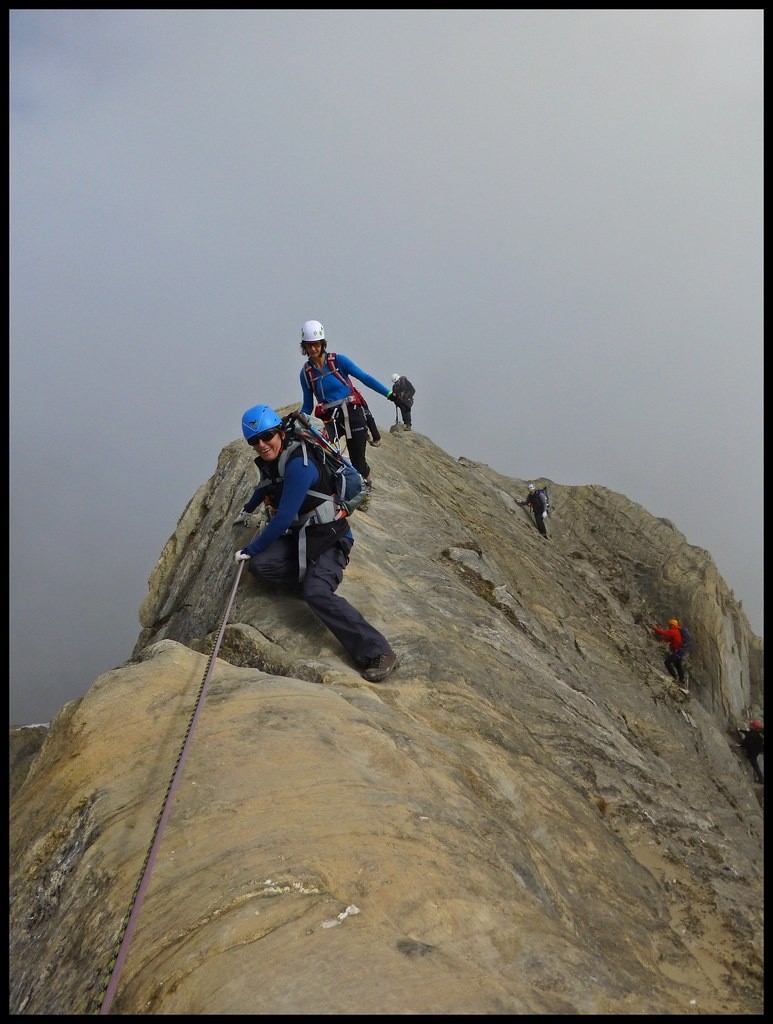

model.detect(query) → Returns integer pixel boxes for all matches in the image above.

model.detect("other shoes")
[404,425,412,431]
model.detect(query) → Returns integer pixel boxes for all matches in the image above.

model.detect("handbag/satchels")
[335,464,363,515]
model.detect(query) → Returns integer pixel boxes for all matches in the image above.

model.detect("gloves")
[515,499,522,504]
[393,392,412,412]
[542,512,547,518]
[234,550,251,567]
[232,507,252,527]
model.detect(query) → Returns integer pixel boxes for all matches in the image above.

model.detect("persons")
[391,373,416,430]
[230,404,401,682]
[297,321,400,486]
[646,619,686,683]
[515,484,548,540]
[737,721,764,785]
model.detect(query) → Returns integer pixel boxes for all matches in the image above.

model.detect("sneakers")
[364,649,399,681]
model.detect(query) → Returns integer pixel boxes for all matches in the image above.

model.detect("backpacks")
[677,627,690,649]
[402,378,416,397]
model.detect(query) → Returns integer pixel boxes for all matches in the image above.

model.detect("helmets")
[752,721,761,729]
[668,619,678,626]
[528,484,535,491]
[301,320,325,343]
[242,404,282,440]
[391,373,401,382]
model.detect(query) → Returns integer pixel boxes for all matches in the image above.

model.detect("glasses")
[247,429,279,446]
[301,340,322,349]
